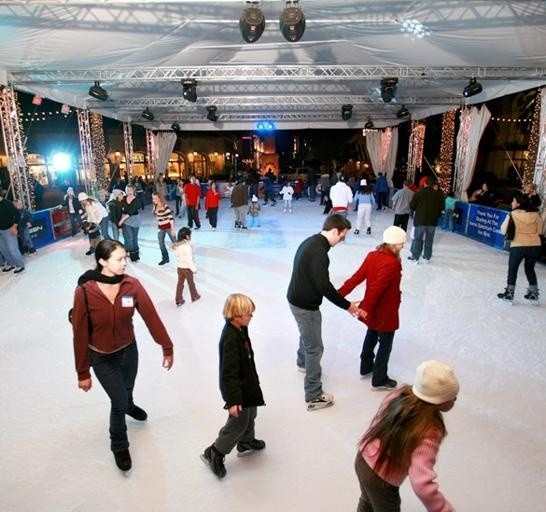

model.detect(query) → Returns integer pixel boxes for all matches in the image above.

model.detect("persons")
[337,225,407,390]
[199,293,266,479]
[392,173,457,261]
[470,181,495,208]
[353,360,460,512]
[70,240,173,471]
[174,227,201,308]
[497,181,545,301]
[0,189,37,273]
[32,177,45,211]
[287,214,361,412]
[153,168,389,241]
[59,172,177,264]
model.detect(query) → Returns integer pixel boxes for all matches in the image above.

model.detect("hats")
[384,226,407,244]
[412,361,459,405]
[78,192,89,201]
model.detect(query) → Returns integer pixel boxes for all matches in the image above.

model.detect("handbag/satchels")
[505,214,515,240]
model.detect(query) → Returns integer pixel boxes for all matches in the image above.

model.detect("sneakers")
[497,289,539,300]
[408,256,429,261]
[306,392,335,403]
[131,253,140,261]
[354,230,372,234]
[361,364,396,387]
[3,266,24,273]
[127,405,147,420]
[86,251,93,255]
[177,296,199,304]
[238,439,265,452]
[205,445,226,477]
[159,260,169,265]
[235,225,247,229]
[115,449,131,471]
